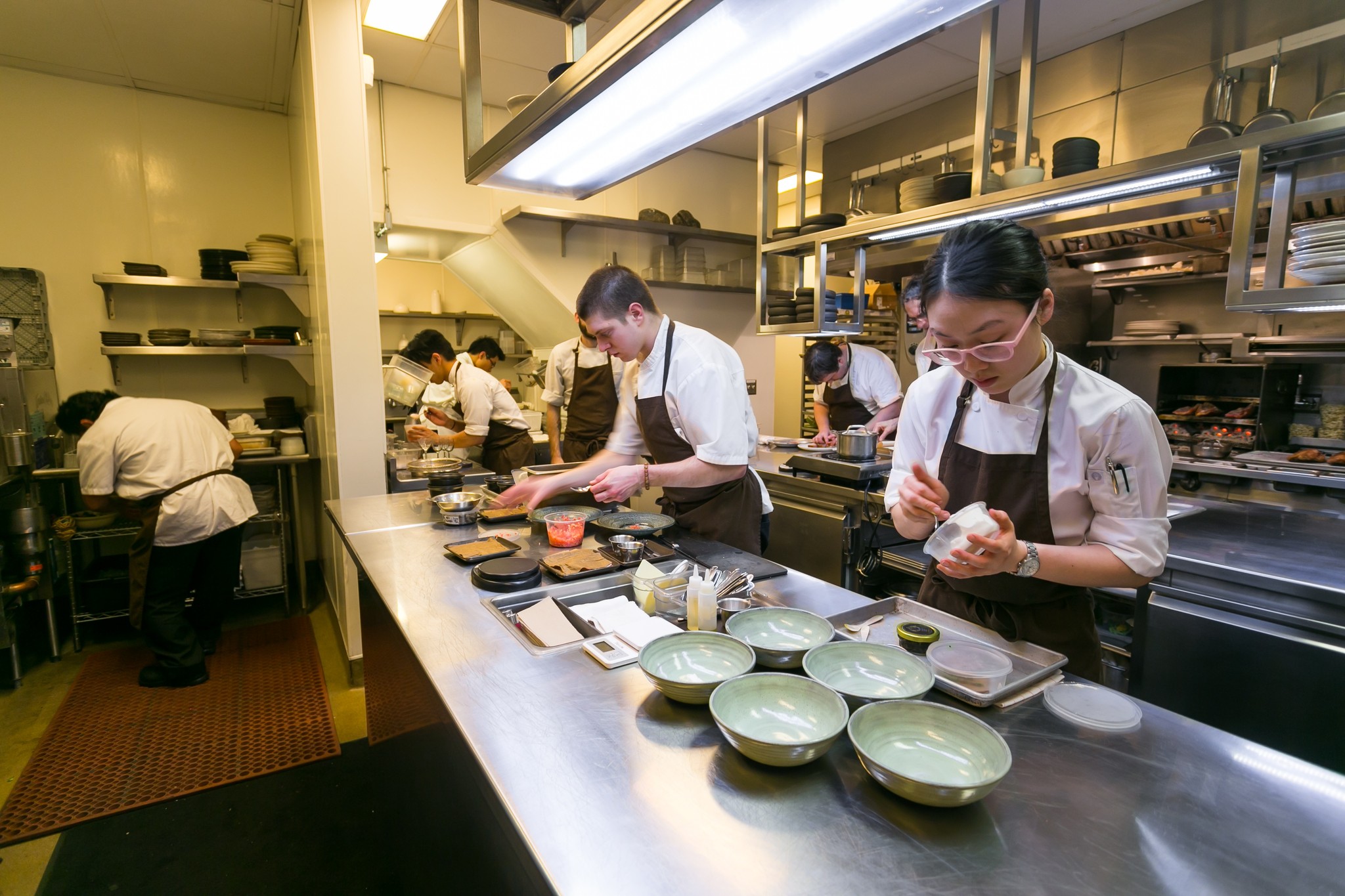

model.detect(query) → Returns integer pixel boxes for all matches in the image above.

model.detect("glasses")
[485,356,497,369]
[920,296,1041,366]
[907,312,927,326]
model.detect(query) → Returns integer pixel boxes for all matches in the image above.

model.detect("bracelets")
[644,463,650,490]
[450,420,455,429]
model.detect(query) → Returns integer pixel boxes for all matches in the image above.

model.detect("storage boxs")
[385,353,435,409]
[498,329,515,355]
[521,409,542,431]
[513,355,542,387]
[241,538,283,590]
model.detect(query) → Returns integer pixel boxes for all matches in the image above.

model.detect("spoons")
[844,615,884,632]
[570,485,592,493]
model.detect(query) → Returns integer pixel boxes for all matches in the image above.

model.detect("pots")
[837,424,879,457]
[1186,53,1344,148]
[933,137,1099,204]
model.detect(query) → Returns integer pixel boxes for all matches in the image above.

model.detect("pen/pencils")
[1106,456,1119,495]
[1116,463,1130,493]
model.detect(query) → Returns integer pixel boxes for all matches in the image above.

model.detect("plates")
[443,504,523,562]
[765,438,837,452]
[251,485,276,516]
[98,326,301,345]
[537,538,676,580]
[1283,220,1345,284]
[1123,321,1178,334]
[122,233,298,281]
[767,174,937,325]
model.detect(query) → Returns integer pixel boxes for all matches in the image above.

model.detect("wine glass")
[418,437,455,458]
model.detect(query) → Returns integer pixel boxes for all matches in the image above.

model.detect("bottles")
[431,288,442,314]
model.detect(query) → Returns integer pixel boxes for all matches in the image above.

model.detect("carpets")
[34,721,537,895]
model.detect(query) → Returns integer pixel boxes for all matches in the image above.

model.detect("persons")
[418,336,512,461]
[882,218,1173,684]
[804,340,905,447]
[873,275,943,442]
[399,329,543,476]
[491,266,773,557]
[540,311,631,510]
[55,389,260,687]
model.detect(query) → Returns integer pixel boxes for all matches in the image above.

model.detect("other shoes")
[138,660,207,686]
[197,626,223,654]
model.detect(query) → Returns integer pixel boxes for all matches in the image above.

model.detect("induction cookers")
[787,448,901,488]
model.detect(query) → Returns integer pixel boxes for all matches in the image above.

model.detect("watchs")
[1006,539,1040,577]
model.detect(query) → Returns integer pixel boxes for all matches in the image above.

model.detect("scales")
[583,616,684,669]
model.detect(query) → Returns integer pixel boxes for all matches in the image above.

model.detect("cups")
[608,535,644,561]
[718,597,752,626]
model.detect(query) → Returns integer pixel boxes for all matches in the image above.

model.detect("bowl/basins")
[71,496,148,529]
[637,499,1002,807]
[405,459,519,525]
[263,395,306,455]
[404,424,428,442]
[528,505,676,548]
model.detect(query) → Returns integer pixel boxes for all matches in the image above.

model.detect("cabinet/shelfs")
[754,110,1345,336]
[503,205,795,297]
[60,465,292,653]
[380,309,530,360]
[92,271,315,389]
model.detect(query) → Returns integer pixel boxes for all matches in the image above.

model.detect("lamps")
[457,0,1000,203]
